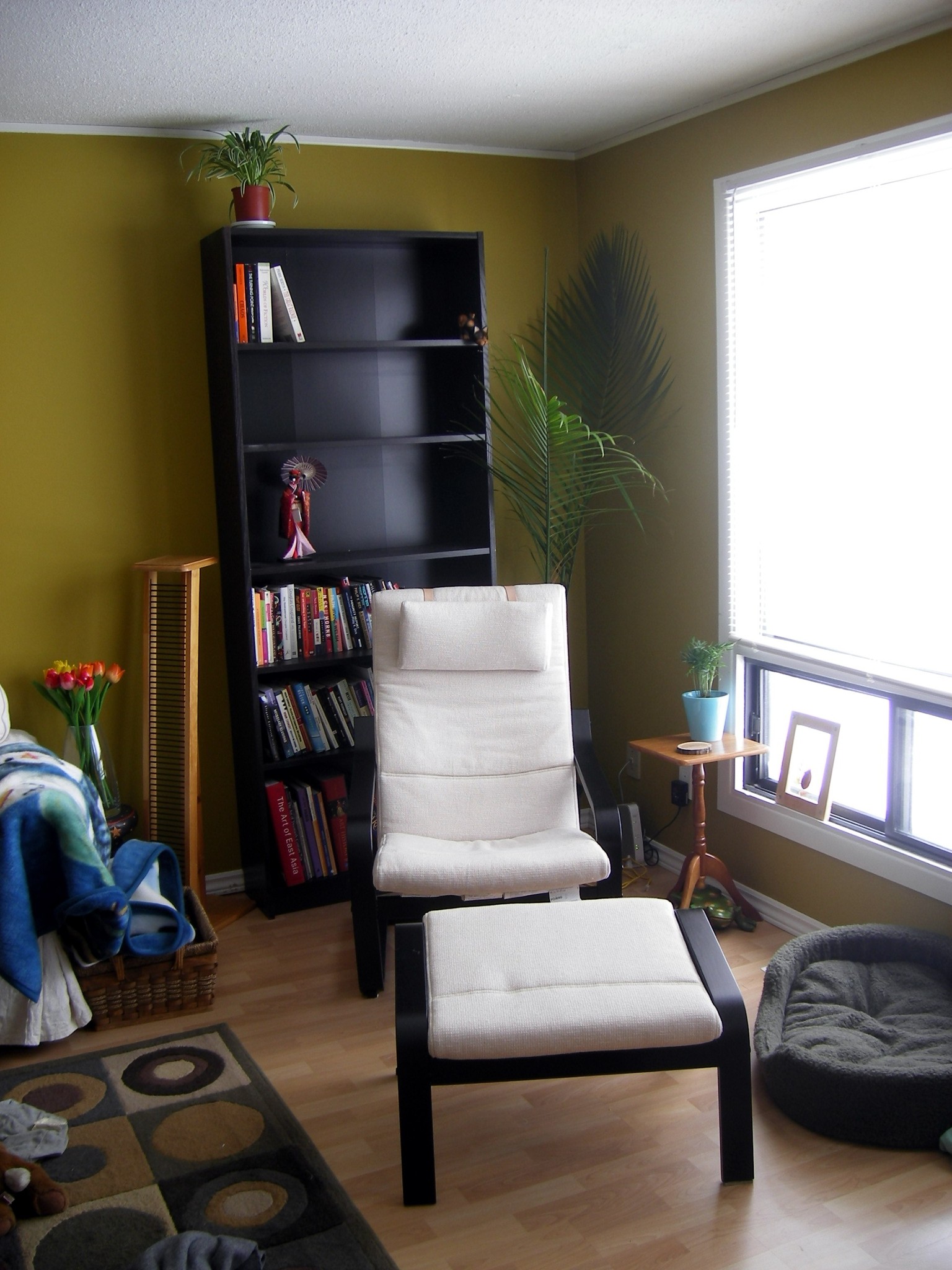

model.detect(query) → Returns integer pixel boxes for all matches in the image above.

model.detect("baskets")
[72,887,219,1030]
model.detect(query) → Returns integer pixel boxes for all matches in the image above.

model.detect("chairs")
[341,581,623,1001]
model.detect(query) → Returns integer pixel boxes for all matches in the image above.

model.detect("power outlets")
[678,764,694,800]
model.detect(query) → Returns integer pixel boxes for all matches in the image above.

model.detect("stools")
[395,897,755,1206]
[105,801,138,854]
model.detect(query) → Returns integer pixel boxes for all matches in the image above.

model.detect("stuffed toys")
[457,310,488,346]
[0,1142,68,1235]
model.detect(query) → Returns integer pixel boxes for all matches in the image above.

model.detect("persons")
[277,470,318,560]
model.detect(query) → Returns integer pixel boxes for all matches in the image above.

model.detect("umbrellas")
[280,455,327,492]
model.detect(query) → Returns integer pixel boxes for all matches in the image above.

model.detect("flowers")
[29,658,125,809]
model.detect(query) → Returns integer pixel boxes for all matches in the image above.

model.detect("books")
[258,666,378,761]
[264,769,348,887]
[233,262,306,344]
[252,576,407,666]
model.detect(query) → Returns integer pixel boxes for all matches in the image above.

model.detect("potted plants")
[180,123,300,219]
[679,636,741,743]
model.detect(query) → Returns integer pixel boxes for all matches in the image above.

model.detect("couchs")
[754,922,952,1151]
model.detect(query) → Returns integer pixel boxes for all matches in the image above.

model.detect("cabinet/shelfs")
[200,224,497,920]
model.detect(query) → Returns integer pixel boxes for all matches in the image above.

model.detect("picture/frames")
[774,710,842,821]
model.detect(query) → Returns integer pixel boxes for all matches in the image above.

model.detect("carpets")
[1,1022,401,1270]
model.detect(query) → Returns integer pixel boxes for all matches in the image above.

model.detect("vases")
[61,721,121,819]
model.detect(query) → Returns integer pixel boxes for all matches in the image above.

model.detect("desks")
[628,731,770,923]
